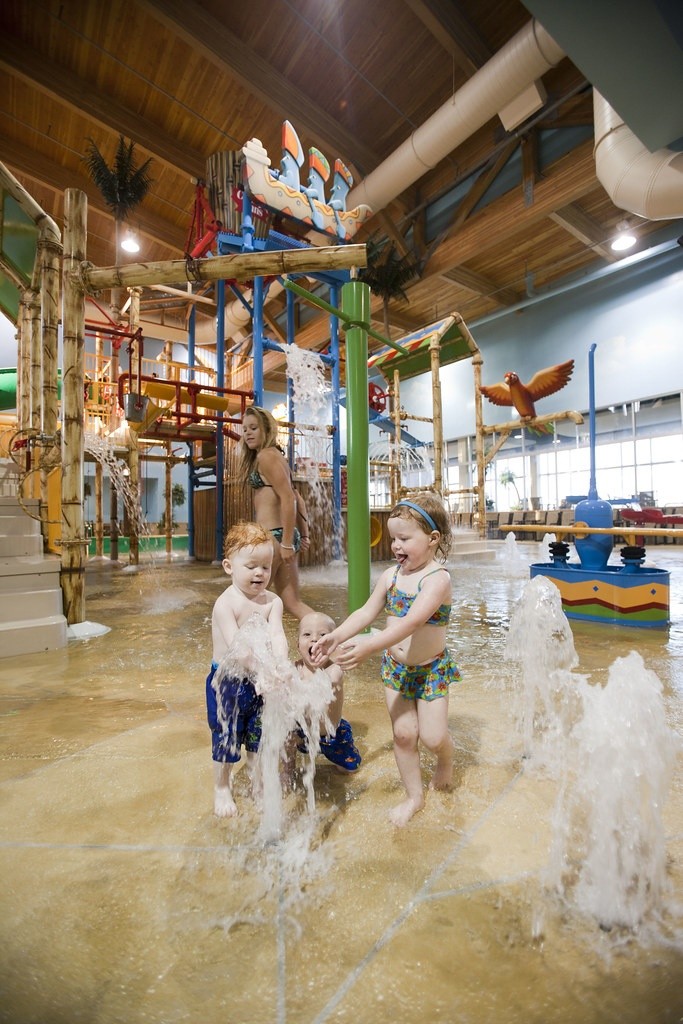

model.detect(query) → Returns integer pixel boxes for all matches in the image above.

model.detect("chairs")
[449,507,683,548]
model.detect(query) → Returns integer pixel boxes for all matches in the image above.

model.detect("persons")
[292,611,362,782]
[205,520,288,820]
[236,407,314,620]
[310,496,460,828]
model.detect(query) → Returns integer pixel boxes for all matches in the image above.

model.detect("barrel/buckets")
[123,393,150,423]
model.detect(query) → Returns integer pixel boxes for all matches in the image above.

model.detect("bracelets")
[300,536,310,539]
[280,544,293,550]
